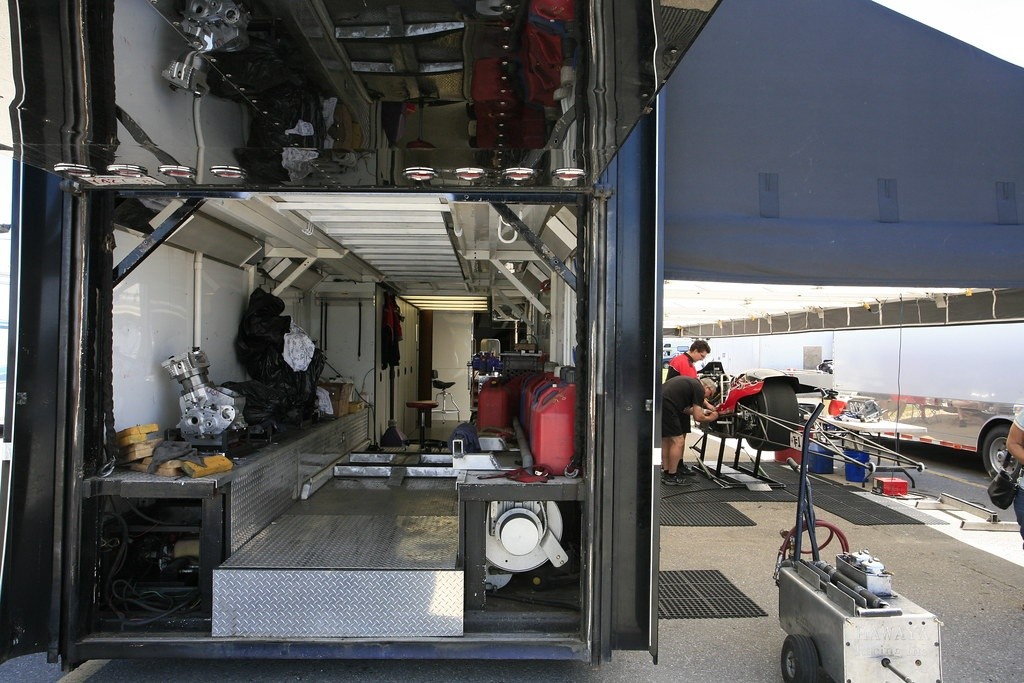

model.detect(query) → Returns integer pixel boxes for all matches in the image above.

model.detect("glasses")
[700,352,705,359]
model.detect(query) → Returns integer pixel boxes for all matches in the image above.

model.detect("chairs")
[432,369,461,423]
[515,343,536,353]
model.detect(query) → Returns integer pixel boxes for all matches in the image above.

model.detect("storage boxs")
[873,477,909,496]
[317,380,354,419]
[835,553,892,597]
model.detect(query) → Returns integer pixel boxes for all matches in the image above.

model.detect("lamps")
[401,101,439,151]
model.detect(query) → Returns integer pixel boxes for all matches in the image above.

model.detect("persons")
[660,340,711,476]
[660,376,719,486]
[1006,409,1024,550]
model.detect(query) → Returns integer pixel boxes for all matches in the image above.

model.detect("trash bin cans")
[843,448,870,483]
[808,442,833,474]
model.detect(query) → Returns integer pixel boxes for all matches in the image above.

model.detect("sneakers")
[661,470,691,486]
[677,459,696,475]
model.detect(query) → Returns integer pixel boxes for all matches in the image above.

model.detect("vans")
[677,345,689,354]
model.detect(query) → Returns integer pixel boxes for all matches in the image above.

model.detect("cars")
[663,353,684,369]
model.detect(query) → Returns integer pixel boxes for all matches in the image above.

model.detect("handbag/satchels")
[987,470,1016,510]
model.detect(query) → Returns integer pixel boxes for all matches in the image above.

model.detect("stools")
[405,400,446,455]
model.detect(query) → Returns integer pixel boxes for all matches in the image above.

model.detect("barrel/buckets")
[477,363,583,477]
[844,449,870,483]
[808,442,834,474]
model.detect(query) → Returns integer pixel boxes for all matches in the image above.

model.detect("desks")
[499,353,540,375]
[803,412,928,489]
